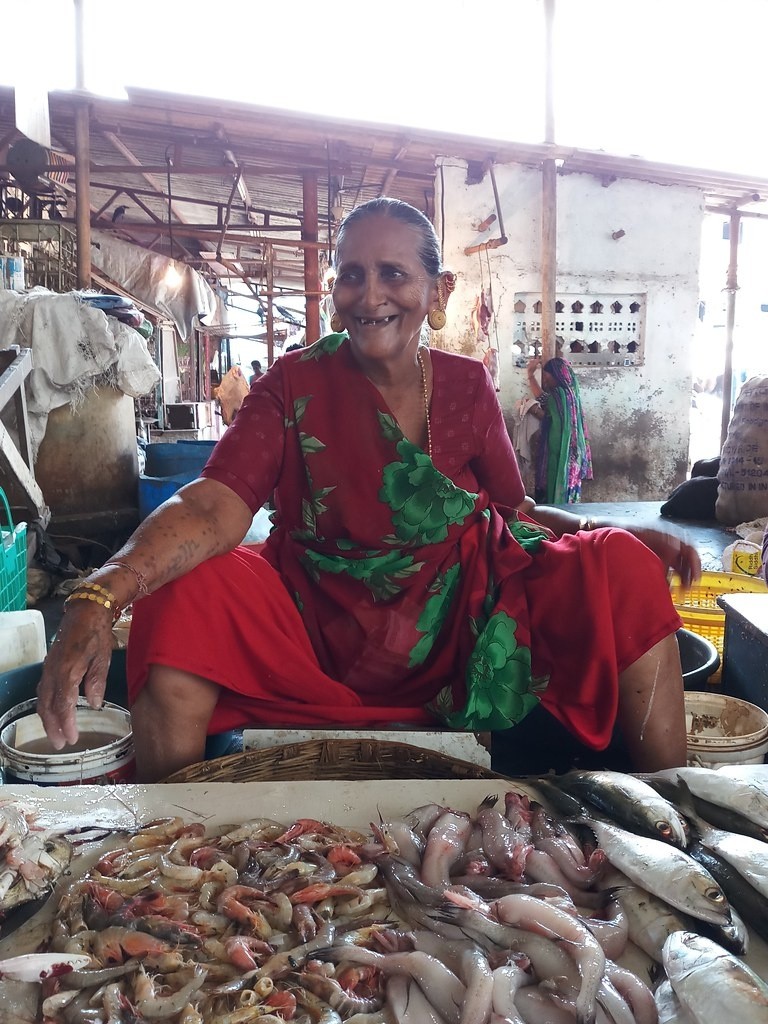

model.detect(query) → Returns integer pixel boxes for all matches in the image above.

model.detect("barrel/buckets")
[684,690,768,766]
[0,695,136,787]
[143,442,215,477]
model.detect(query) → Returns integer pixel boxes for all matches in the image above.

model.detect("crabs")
[0,800,64,906]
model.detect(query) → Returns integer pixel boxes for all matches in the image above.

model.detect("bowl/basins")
[676,628,721,692]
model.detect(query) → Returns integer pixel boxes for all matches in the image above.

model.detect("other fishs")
[307,770,766,1024]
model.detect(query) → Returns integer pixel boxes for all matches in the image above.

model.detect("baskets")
[659,568,767,685]
[159,739,511,779]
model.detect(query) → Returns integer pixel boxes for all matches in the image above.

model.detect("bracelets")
[103,561,144,607]
[534,407,538,414]
[79,583,122,618]
[529,376,534,379]
[580,513,597,530]
[63,593,116,619]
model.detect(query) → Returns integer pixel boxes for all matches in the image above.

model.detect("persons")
[37,197,702,783]
[528,358,594,504]
[250,361,265,386]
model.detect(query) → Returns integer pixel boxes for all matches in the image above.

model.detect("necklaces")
[417,351,433,460]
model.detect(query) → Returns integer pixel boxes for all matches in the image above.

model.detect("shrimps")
[35,818,397,1024]
